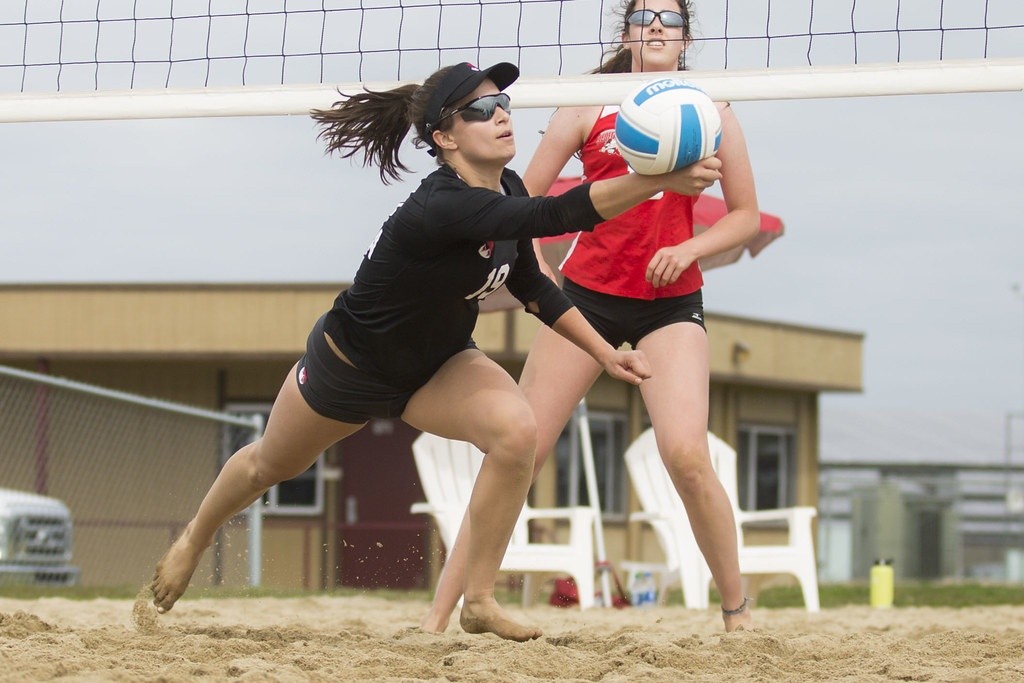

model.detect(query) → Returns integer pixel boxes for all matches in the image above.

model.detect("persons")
[149,60,721,643]
[426,0,760,634]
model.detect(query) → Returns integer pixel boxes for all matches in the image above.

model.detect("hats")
[423,62,520,157]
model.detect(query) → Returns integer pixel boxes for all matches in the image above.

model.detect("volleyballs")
[614,77,723,175]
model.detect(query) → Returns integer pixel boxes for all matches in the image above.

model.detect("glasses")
[430,92,511,130]
[626,10,685,27]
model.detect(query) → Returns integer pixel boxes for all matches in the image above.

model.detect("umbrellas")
[479,174,782,606]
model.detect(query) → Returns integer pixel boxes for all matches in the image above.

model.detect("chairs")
[411,432,595,610]
[623,424,821,611]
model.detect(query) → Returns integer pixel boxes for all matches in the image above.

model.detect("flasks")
[870,559,896,609]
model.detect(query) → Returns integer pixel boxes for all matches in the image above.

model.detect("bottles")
[627,562,656,611]
